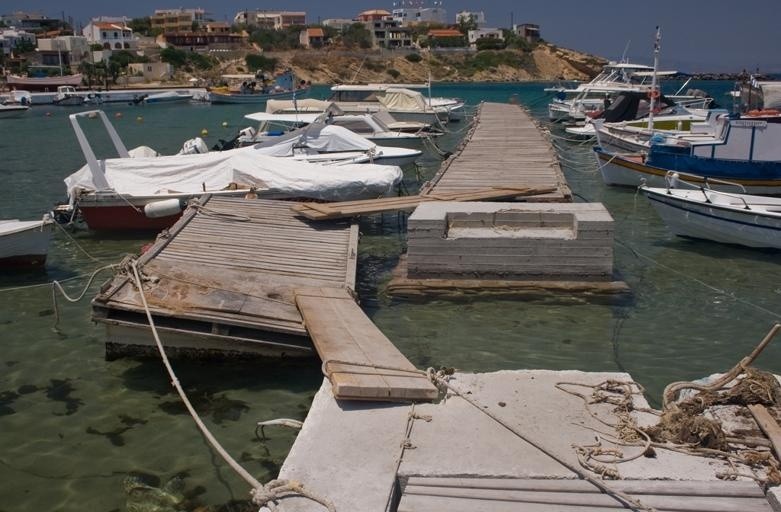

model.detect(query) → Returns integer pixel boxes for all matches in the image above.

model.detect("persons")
[239,80,312,95]
[210,138,226,150]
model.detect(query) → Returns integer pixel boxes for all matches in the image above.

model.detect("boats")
[214,73,466,179]
[0,216,55,274]
[0,90,33,111]
[52,86,86,107]
[544,25,781,249]
[128,90,193,107]
[82,92,105,107]
[208,70,311,105]
[52,109,405,232]
